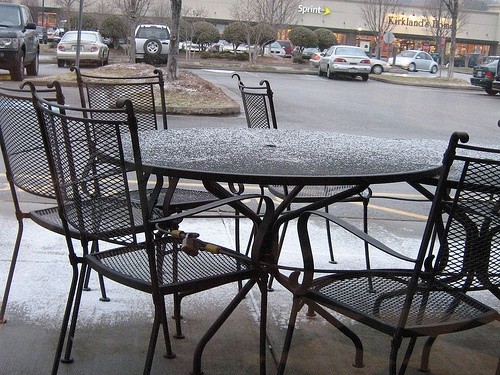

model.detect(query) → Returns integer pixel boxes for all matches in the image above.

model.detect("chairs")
[159,33,166,39]
[0,73,500,375]
[140,32,147,37]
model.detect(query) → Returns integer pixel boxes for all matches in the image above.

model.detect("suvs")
[0,2,40,81]
[277,39,295,58]
[470,56,500,96]
[133,24,170,64]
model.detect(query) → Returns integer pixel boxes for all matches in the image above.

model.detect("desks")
[92,128,444,375]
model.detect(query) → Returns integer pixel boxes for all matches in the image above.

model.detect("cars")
[302,45,391,81]
[179,40,259,54]
[36,26,113,67]
[388,49,439,73]
[262,41,286,58]
